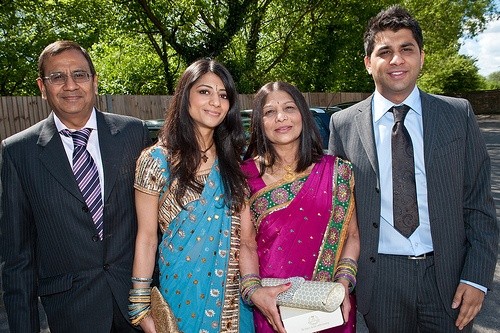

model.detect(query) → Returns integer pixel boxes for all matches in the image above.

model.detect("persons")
[0,41,153,333]
[240,80,360,333]
[128,59,255,333]
[328,4,500,333]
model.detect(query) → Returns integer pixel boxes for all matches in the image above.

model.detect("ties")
[387,105,421,239]
[58,129,103,240]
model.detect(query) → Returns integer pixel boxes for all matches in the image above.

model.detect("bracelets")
[128,277,153,326]
[241,273,263,306]
[333,257,358,293]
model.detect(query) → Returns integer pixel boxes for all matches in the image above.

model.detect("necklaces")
[200,140,215,163]
[281,160,297,182]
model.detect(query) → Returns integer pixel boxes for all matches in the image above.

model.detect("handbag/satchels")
[261,276,346,312]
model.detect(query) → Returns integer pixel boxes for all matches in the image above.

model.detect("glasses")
[42,72,93,85]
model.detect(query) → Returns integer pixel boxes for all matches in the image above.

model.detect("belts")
[378,251,434,260]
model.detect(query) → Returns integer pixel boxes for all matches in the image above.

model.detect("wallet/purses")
[151,286,180,333]
